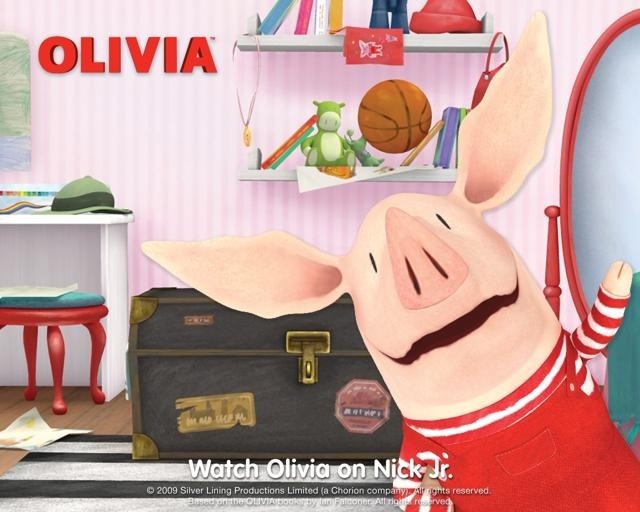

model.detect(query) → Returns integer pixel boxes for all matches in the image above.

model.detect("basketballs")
[357,79,432,153]
